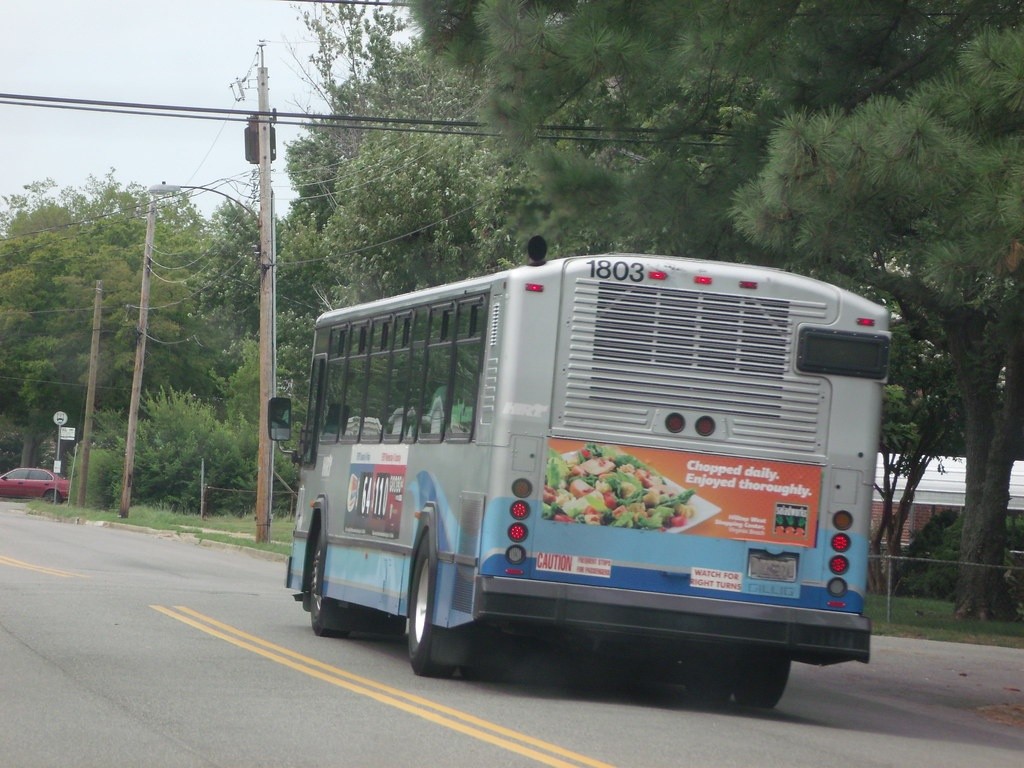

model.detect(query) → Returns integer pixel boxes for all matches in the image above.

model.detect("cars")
[0,468,71,504]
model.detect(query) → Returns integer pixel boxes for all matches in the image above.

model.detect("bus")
[268,236,893,712]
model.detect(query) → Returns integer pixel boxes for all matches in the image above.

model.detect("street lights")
[149,185,277,543]
